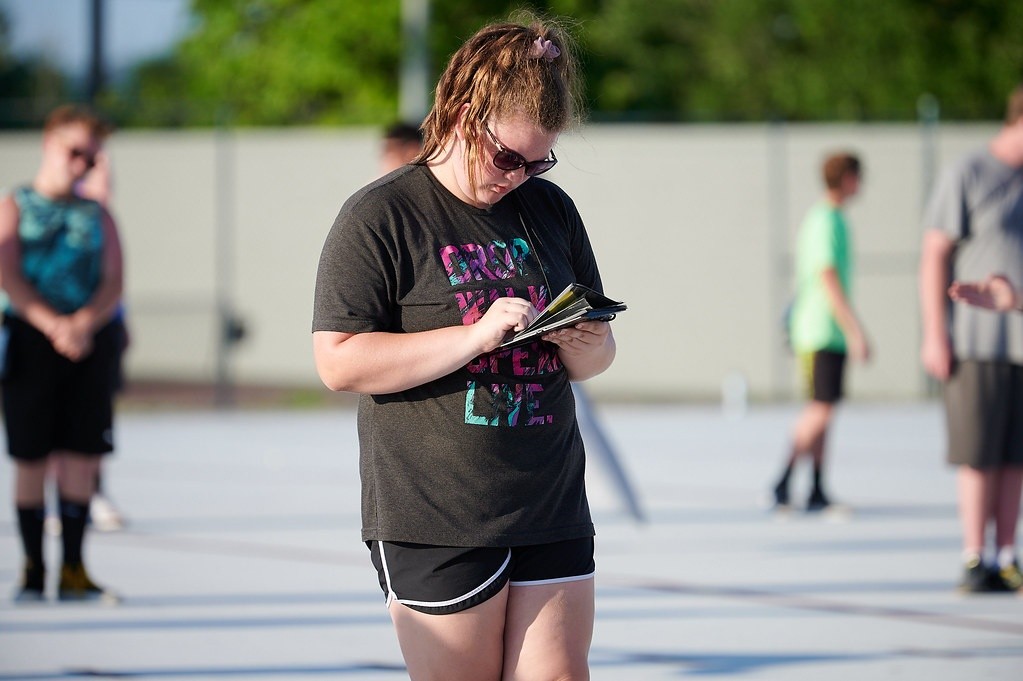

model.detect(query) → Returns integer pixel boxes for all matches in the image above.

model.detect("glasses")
[52,139,96,170]
[477,117,558,176]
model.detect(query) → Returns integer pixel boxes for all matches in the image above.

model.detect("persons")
[919,82,1023,596]
[770,154,871,513]
[0,102,129,608]
[380,120,427,175]
[947,272,1023,315]
[308,24,617,681]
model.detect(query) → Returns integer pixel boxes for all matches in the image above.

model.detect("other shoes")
[59,571,111,601]
[961,561,990,592]
[15,569,47,604]
[806,493,838,513]
[989,559,1023,593]
[775,483,791,505]
